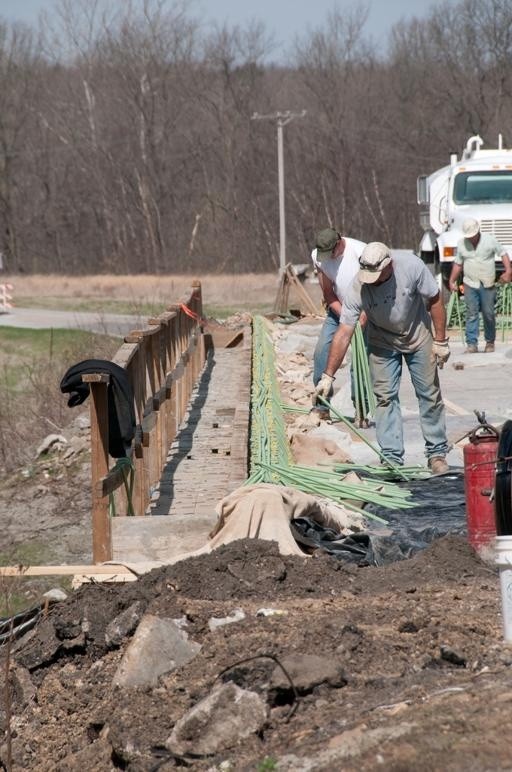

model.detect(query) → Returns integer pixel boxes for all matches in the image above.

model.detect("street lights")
[249,109,309,271]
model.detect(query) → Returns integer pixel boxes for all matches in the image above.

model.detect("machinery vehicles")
[412,131,512,309]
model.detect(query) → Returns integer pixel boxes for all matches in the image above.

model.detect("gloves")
[312,371,336,407]
[432,340,450,369]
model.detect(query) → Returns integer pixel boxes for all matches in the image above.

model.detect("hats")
[463,219,480,238]
[316,229,339,262]
[356,242,392,284]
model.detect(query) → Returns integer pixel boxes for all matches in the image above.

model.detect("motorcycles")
[285,263,311,283]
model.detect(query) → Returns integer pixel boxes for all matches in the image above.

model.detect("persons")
[448,217,511,354]
[307,227,370,428]
[315,240,452,476]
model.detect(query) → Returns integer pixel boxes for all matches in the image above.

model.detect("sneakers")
[465,343,495,353]
[428,456,449,474]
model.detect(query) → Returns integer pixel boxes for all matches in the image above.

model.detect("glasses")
[358,256,390,271]
